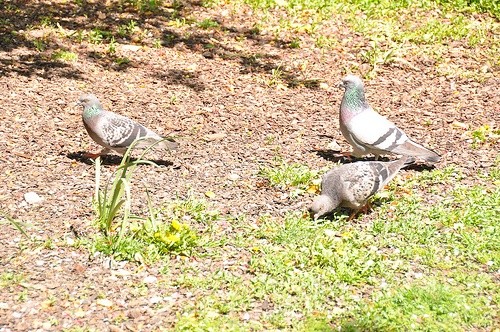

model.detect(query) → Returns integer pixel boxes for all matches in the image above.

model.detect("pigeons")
[75,95,180,160]
[312,156,405,222]
[339,76,441,161]
